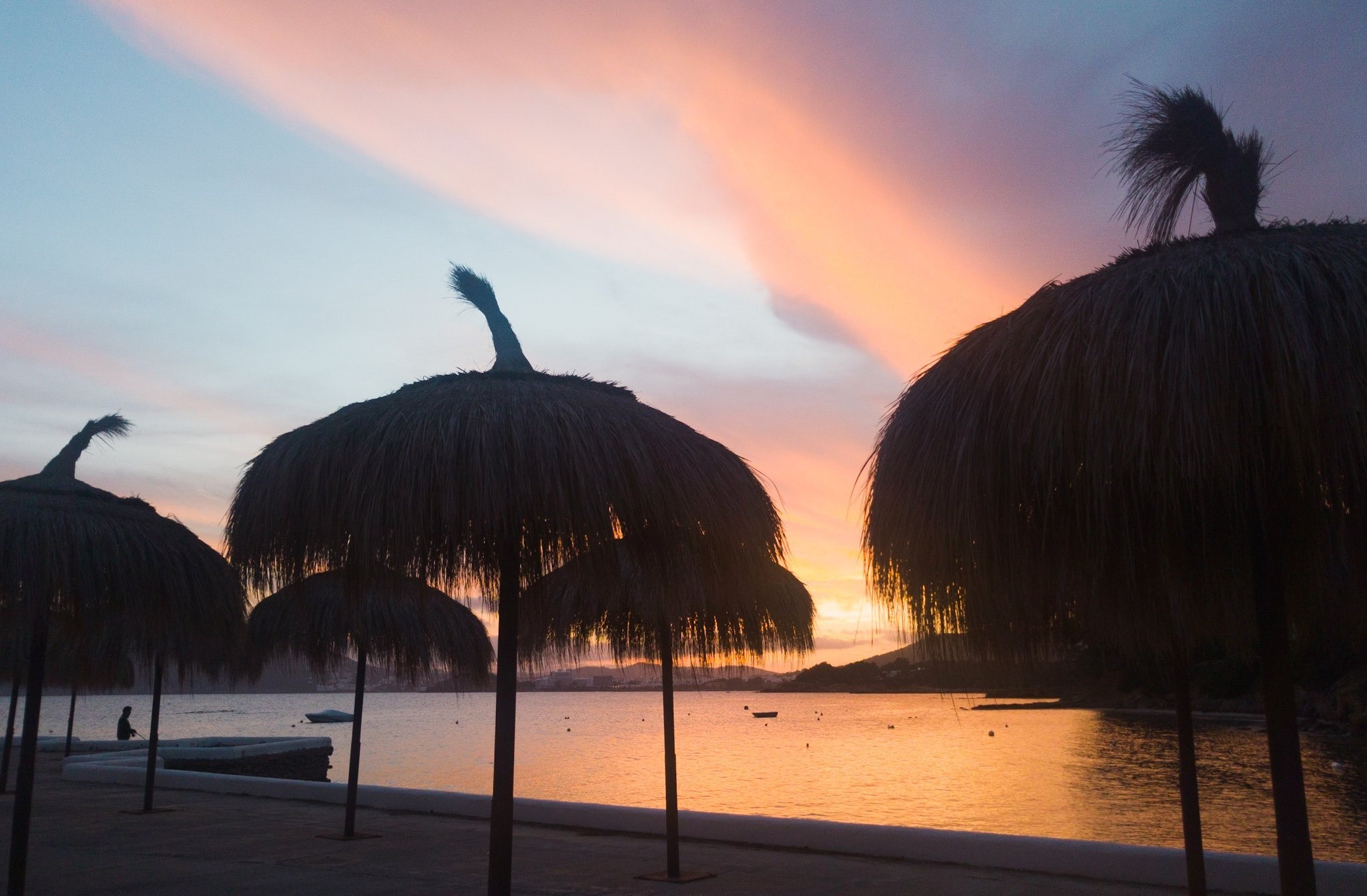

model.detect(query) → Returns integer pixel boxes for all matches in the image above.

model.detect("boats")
[305,709,354,723]
[751,711,778,718]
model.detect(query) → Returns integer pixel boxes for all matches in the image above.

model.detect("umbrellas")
[226,269,790,896]
[856,69,1367,896]
[0,415,250,896]
[512,524,816,896]
[232,562,496,839]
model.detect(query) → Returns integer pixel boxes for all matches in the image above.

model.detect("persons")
[117,707,137,741]
